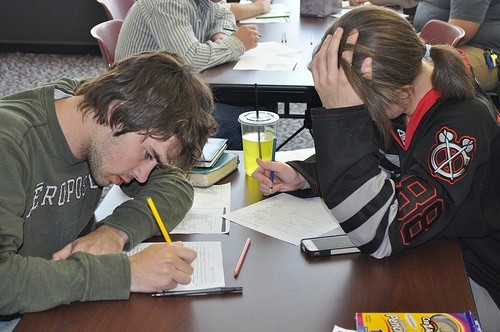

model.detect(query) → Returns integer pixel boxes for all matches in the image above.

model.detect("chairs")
[96,0,136,20]
[420,19,466,46]
[90,20,125,65]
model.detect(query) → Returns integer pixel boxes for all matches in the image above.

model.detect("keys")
[485,49,498,70]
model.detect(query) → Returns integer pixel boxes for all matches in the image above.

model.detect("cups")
[237,111,279,176]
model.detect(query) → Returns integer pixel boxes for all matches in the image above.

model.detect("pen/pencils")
[311,38,313,45]
[223,28,262,38]
[148,197,173,243]
[234,237,251,278]
[256,16,290,19]
[269,139,277,193]
[151,287,243,296]
[281,32,287,43]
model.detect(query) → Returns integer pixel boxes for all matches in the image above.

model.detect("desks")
[12,150,482,332]
[201,0,403,96]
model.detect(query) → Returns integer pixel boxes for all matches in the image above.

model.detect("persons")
[210,1,272,20]
[255,4,500,307]
[113,0,260,76]
[410,0,500,91]
[1,50,220,318]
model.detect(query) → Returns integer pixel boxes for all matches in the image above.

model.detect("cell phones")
[300,234,362,258]
